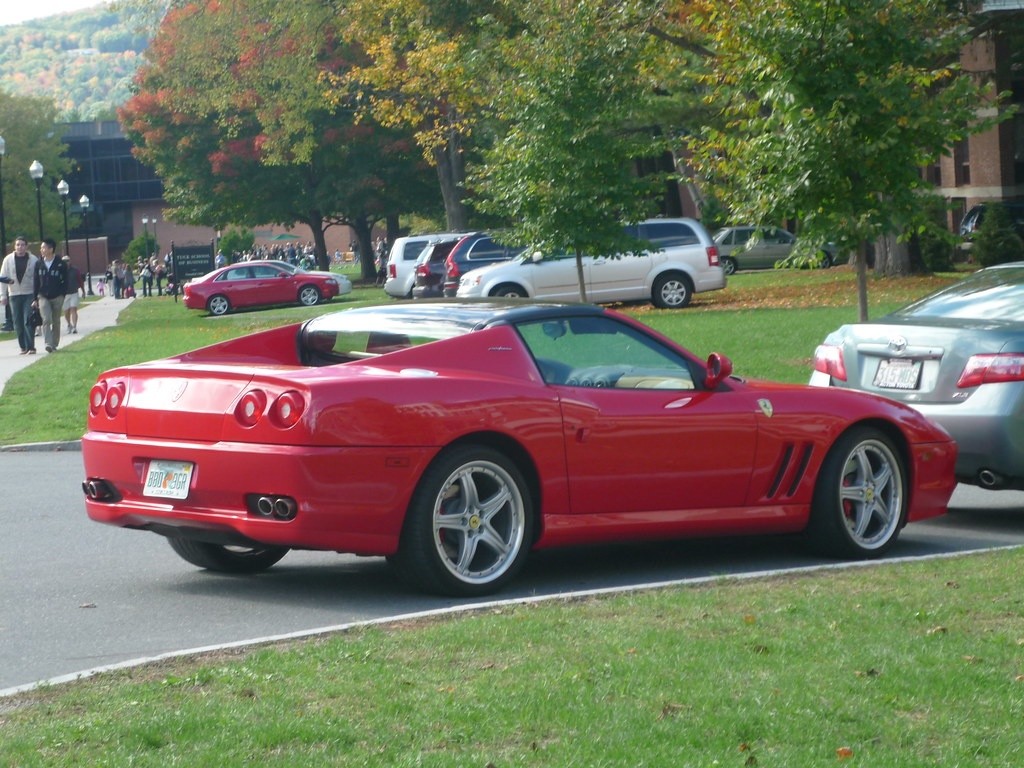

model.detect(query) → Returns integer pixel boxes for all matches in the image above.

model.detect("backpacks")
[160,265,167,275]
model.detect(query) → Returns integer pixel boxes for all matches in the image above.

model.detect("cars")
[808,261,1024,491]
[80,297,958,596]
[183,260,352,315]
[712,227,875,275]
[959,202,1024,241]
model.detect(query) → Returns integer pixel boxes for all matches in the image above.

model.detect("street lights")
[142,218,149,258]
[57,179,70,256]
[152,219,157,252]
[0,136,7,272]
[79,195,94,295]
[29,160,43,243]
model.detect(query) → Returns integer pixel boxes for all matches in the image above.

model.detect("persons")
[0,238,87,355]
[96,235,389,299]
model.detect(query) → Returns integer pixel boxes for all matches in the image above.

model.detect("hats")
[62,255,71,261]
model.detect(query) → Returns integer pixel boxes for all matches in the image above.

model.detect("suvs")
[384,217,728,309]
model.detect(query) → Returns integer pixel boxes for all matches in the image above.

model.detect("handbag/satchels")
[140,264,152,278]
[26,308,42,328]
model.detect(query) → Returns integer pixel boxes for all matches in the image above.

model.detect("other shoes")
[73,327,77,333]
[28,349,36,354]
[45,346,57,353]
[67,325,72,334]
[20,349,27,354]
[1,323,14,331]
